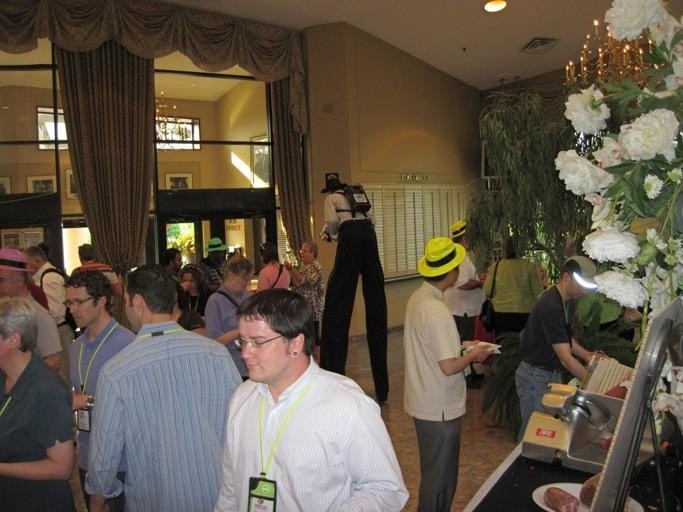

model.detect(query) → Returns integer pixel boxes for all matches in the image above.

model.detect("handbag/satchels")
[480,300,497,331]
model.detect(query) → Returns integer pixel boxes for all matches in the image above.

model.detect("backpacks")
[333,185,371,212]
[41,267,86,339]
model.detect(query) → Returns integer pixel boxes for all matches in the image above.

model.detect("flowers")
[555,1,683,396]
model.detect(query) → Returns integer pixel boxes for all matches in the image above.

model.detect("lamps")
[563,18,663,86]
[154,57,191,144]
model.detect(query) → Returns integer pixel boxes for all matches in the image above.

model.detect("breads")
[542,487,578,512]
[580,473,601,507]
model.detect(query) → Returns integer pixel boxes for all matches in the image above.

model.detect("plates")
[531,482,644,512]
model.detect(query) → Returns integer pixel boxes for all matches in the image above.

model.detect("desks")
[461,375,682,512]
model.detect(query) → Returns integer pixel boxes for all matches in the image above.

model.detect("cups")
[283,253,291,263]
[250,279,258,290]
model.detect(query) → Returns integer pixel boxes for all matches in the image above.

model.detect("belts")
[536,364,565,372]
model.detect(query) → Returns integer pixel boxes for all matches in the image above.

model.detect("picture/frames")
[0,176,12,194]
[66,169,78,198]
[27,175,57,193]
[250,134,270,188]
[0,227,44,251]
[166,174,193,189]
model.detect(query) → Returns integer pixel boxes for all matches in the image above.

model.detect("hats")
[418,238,466,277]
[0,249,35,271]
[204,238,227,252]
[450,220,466,238]
[563,256,597,284]
[321,178,347,193]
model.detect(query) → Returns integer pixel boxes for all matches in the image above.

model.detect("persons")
[0,295,79,512]
[84,261,244,512]
[62,269,138,511]
[404,235,492,511]
[213,287,410,511]
[515,256,610,442]
[1,241,325,404]
[483,233,544,357]
[320,173,390,404]
[538,266,553,289]
[444,220,488,390]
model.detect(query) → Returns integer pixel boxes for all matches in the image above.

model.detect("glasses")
[63,296,94,307]
[235,335,282,347]
